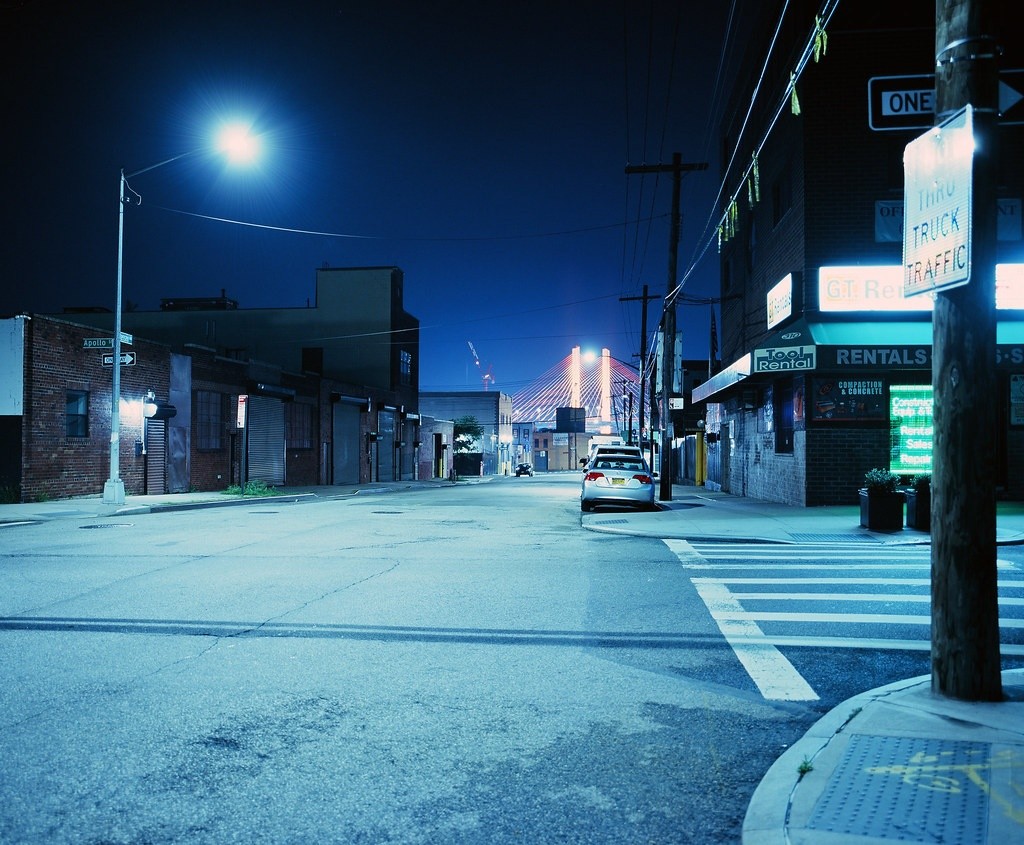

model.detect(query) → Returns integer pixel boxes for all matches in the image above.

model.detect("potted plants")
[905,473,930,527]
[858,467,905,530]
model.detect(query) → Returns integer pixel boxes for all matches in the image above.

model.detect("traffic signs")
[102,351,136,367]
[867,66,1023,133]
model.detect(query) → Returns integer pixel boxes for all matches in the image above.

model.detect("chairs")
[629,465,638,469]
[601,462,611,468]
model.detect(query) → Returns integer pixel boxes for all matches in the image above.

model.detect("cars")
[515,462,534,477]
[579,454,658,512]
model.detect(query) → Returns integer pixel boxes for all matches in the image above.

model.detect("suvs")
[586,435,643,469]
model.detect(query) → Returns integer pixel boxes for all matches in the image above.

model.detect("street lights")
[110,126,261,481]
